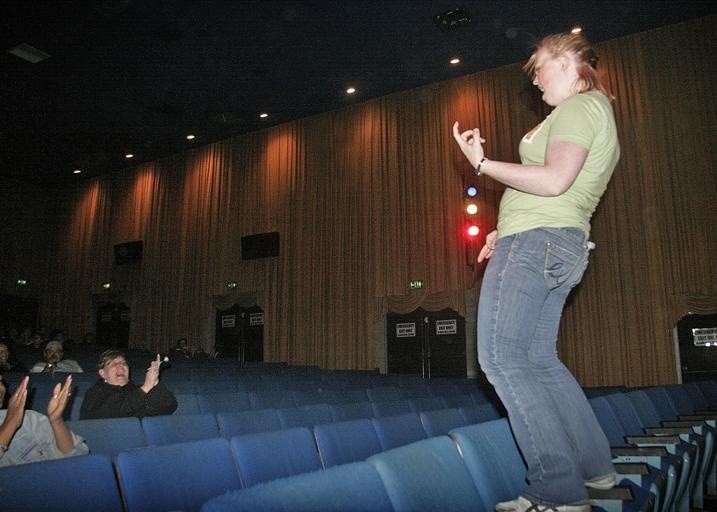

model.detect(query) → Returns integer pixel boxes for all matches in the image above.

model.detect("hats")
[43,340,64,353]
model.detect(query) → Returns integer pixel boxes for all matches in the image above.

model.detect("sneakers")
[494,495,592,512]
[583,471,617,490]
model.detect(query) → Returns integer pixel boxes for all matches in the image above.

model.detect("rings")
[68,392,71,396]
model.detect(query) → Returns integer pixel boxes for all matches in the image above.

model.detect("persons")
[78,348,178,420]
[174,337,192,362]
[450,29,623,512]
[0,318,174,359]
[0,336,29,373]
[26,338,84,374]
[0,364,90,469]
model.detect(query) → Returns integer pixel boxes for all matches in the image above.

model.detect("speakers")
[241,231,280,261]
[114,242,143,264]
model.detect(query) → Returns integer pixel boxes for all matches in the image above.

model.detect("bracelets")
[471,155,489,177]
[46,361,53,368]
